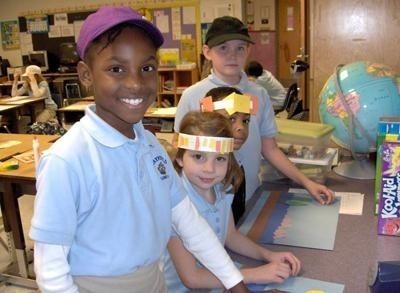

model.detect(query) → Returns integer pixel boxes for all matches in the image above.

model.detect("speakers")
[18,16,27,32]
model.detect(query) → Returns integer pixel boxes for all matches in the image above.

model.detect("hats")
[205,15,256,49]
[22,65,41,76]
[76,6,165,60]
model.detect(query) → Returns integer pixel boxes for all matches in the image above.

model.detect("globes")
[319,61,400,179]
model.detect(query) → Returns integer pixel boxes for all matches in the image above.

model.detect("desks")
[0,67,400,289]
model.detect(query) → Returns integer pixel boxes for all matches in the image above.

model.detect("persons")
[28,8,288,293]
[11,65,58,124]
[200,87,250,226]
[165,111,301,291]
[245,61,287,112]
[174,17,333,205]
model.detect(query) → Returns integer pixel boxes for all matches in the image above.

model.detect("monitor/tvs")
[28,51,49,74]
[59,42,81,67]
